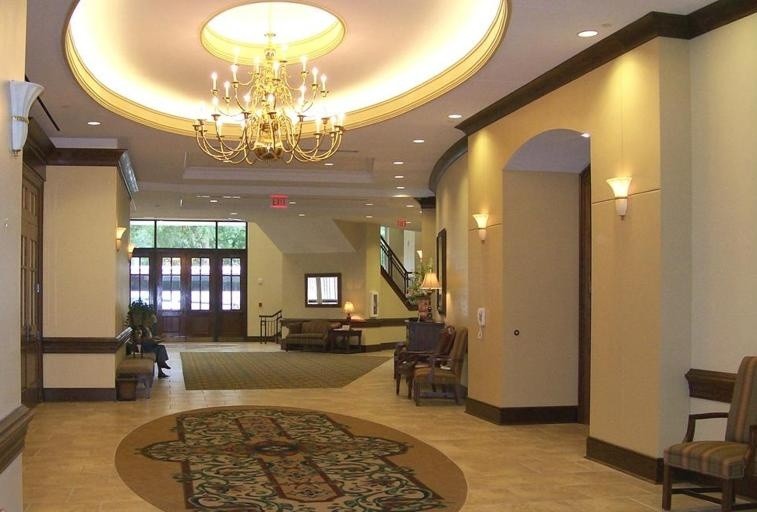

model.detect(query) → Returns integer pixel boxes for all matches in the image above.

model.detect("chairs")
[394,326,468,406]
[662,356,757,512]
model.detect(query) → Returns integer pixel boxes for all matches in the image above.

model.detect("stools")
[117,351,156,399]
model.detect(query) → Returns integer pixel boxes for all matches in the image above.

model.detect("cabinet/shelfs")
[404,320,445,351]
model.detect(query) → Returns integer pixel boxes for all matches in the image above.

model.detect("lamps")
[472,214,488,243]
[419,269,442,323]
[343,301,355,327]
[128,244,135,262]
[10,80,44,159]
[192,6,347,165]
[116,226,127,252]
[416,249,422,262]
[606,176,632,221]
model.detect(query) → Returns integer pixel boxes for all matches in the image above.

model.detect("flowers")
[405,257,433,304]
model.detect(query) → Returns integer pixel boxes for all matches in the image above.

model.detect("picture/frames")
[436,228,446,317]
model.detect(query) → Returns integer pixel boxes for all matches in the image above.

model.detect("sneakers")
[158,373,169,377]
[161,363,170,369]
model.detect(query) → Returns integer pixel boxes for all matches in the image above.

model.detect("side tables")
[329,328,362,354]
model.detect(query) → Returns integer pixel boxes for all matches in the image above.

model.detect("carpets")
[115,405,468,512]
[179,352,394,390]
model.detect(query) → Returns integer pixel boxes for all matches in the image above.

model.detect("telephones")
[476,308,486,326]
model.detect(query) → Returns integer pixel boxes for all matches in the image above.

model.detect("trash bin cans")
[116,375,138,401]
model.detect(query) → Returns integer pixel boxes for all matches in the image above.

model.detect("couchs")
[284,320,341,353]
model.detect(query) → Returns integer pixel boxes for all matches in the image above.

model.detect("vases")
[415,296,430,322]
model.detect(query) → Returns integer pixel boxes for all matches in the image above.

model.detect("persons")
[134,314,172,378]
